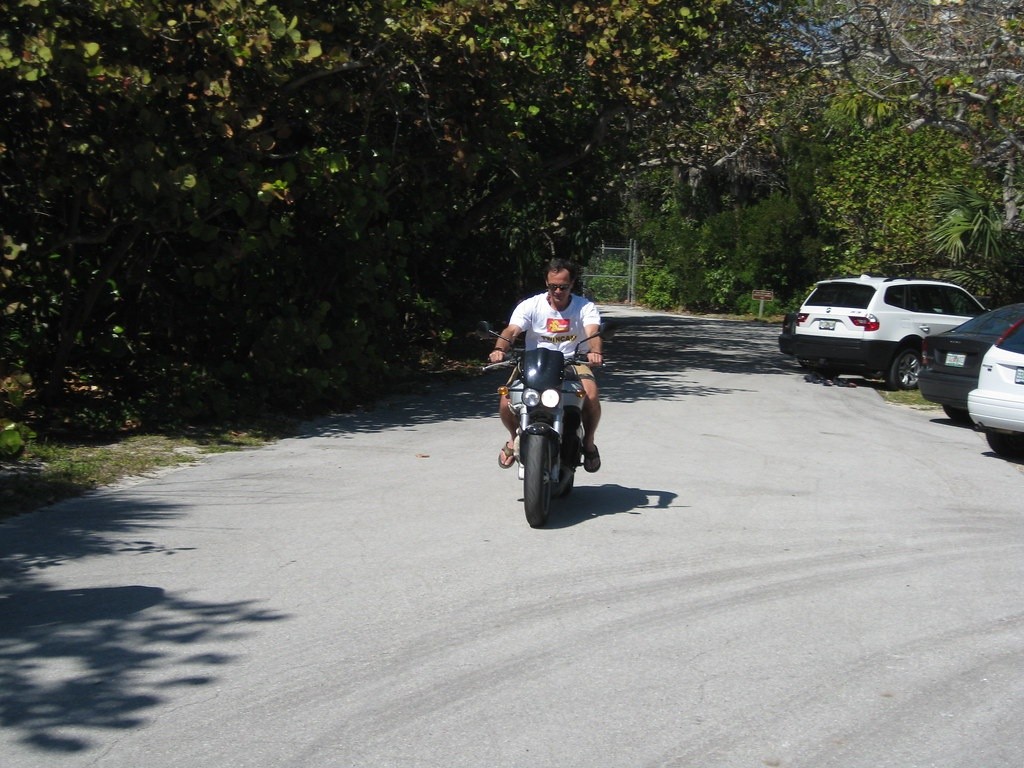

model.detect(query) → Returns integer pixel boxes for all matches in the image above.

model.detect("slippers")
[583,444,600,473]
[498,441,515,469]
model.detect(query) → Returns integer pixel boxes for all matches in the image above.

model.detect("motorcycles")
[479,319,617,528]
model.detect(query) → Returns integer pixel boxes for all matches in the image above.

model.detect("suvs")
[791,272,992,392]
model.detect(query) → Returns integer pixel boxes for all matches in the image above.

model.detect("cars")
[779,308,799,356]
[967,316,1024,459]
[916,302,1024,421]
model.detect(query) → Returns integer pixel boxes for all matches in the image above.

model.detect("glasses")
[547,277,571,291]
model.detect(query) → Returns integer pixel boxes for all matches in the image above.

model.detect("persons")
[489,261,602,473]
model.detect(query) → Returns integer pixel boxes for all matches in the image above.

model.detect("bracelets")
[493,346,504,352]
